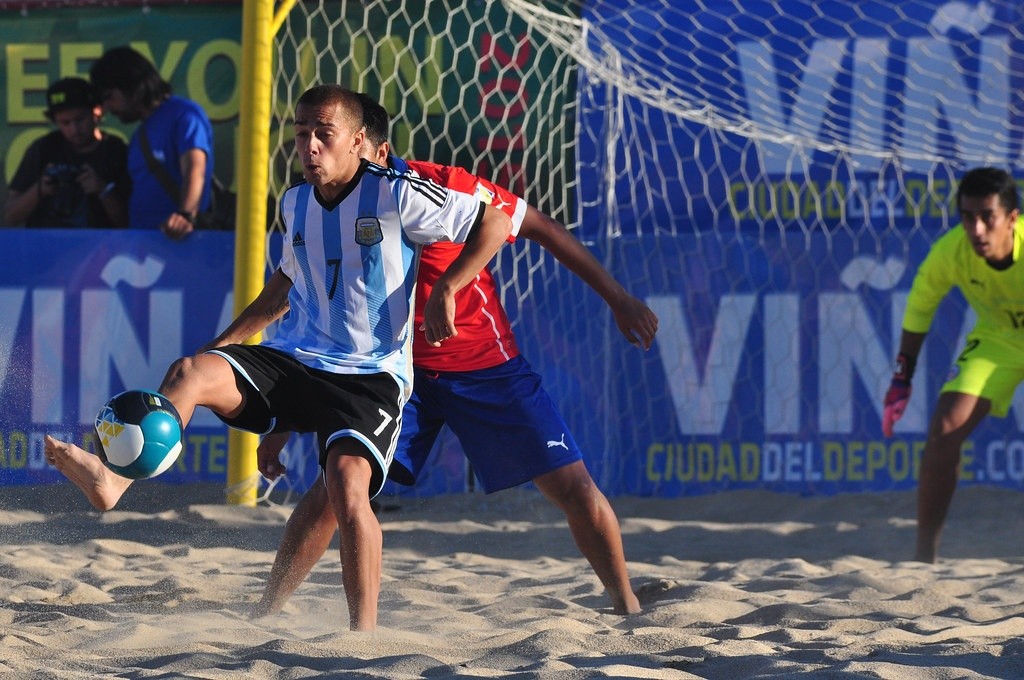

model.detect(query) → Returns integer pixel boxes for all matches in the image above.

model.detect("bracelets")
[175,209,198,230]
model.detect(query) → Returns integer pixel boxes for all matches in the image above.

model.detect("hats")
[42,77,90,119]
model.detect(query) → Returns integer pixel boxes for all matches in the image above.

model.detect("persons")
[882,166,1024,565]
[41,84,663,636]
[1,45,215,237]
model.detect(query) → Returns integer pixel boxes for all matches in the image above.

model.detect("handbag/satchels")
[195,175,238,231]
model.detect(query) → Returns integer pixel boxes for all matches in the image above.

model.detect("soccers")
[92,386,186,482]
